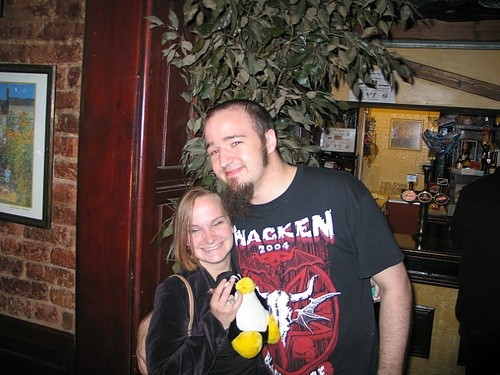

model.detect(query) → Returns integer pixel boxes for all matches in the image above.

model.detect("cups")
[464,119,472,124]
[381,181,407,196]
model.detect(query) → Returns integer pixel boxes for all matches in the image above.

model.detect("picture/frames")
[390,118,424,152]
[0,63,57,230]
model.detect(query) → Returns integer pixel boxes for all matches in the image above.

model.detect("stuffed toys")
[216,272,281,358]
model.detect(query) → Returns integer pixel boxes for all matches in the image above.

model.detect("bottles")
[455,154,470,169]
[480,144,496,175]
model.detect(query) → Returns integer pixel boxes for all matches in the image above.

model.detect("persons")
[452,166,500,375]
[146,187,265,375]
[136,99,412,375]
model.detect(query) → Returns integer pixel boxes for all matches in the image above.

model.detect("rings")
[227,299,234,304]
